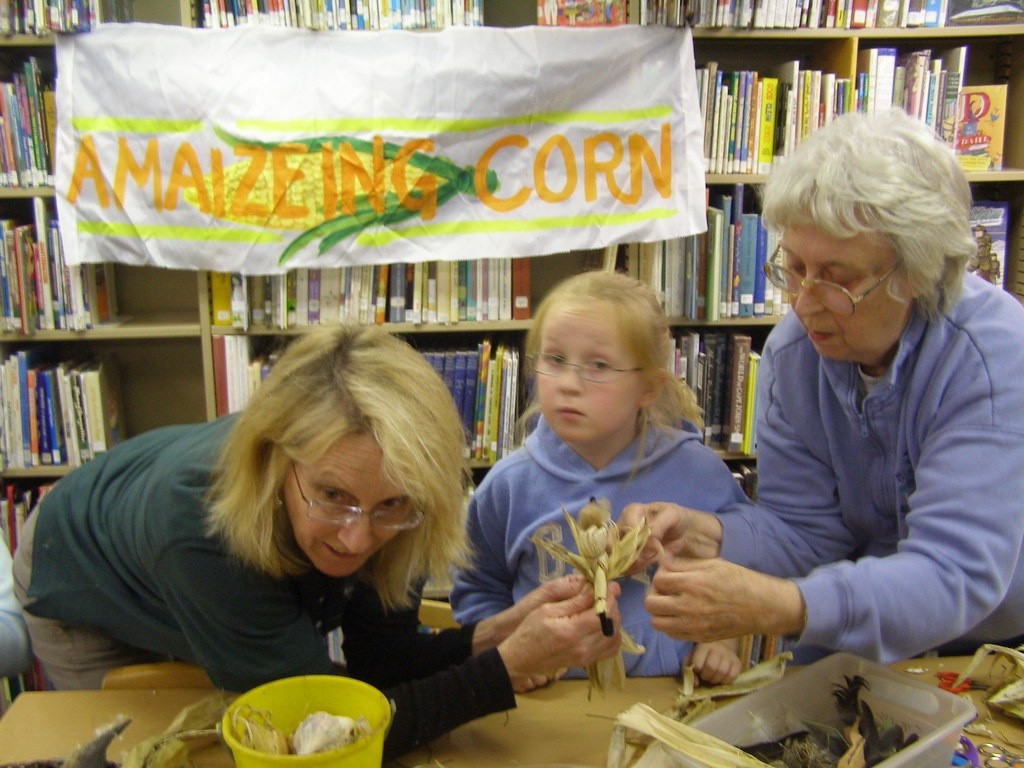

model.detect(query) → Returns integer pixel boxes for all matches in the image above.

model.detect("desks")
[0,654,1023,768]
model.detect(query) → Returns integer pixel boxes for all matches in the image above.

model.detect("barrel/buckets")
[216,674,397,768]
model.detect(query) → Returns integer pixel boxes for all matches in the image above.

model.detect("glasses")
[525,352,643,382]
[763,245,903,316]
[291,462,424,528]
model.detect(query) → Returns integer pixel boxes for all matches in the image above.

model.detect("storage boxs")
[667,652,977,768]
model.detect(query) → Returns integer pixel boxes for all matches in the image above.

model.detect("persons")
[12,322,620,767]
[604,108,1023,665]
[0,527,32,680]
[446,269,755,694]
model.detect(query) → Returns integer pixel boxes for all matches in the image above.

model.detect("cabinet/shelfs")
[0,0,1023,698]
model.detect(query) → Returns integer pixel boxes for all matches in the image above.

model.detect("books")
[192,0,1014,489]
[0,1,132,548]
